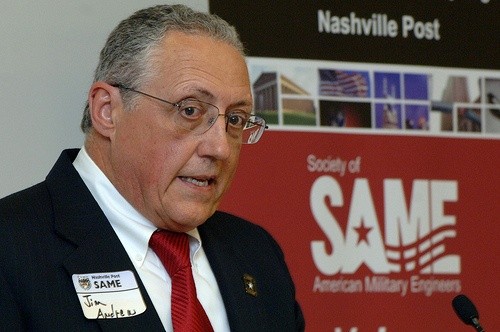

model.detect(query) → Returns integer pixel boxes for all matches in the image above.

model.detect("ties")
[148,230,215,332]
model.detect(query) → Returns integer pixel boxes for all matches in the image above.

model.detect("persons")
[0,4,306,332]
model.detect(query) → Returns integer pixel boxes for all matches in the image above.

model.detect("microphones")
[451,293,486,332]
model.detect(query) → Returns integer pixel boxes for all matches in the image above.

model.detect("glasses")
[109,83,269,144]
